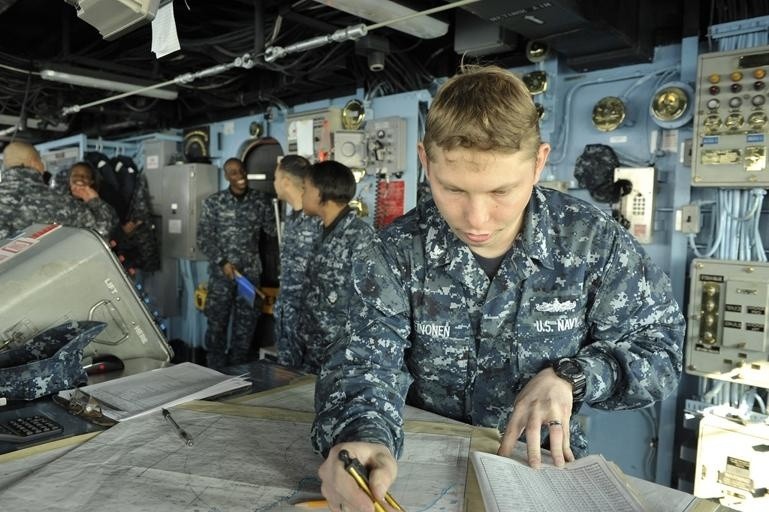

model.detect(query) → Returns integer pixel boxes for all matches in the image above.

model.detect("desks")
[0,362,721,512]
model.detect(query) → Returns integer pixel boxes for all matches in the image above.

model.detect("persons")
[67,162,142,249]
[309,66,686,511]
[270,155,312,370]
[193,157,280,367]
[295,160,377,376]
[1,141,120,239]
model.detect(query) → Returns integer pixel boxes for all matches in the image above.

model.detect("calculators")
[0,414,63,443]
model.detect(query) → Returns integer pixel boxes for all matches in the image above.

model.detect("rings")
[548,419,561,427]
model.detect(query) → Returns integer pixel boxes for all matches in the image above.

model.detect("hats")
[0,321,107,400]
[574,144,619,201]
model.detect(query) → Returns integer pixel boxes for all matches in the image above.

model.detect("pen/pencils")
[291,501,328,508]
[162,406,193,446]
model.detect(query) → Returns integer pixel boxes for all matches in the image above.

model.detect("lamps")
[354,34,390,73]
[315,0,450,40]
[41,70,181,104]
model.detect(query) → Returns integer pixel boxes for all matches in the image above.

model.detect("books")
[471,450,649,511]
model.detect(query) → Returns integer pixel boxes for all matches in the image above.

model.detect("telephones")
[612,167,654,245]
